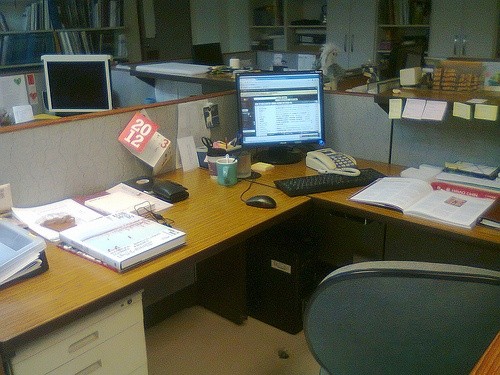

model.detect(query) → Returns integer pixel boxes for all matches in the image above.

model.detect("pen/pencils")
[226,154,230,164]
[232,152,241,163]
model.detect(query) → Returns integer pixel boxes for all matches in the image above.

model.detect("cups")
[233,151,251,178]
[143,97,155,104]
[230,59,240,69]
[216,157,238,186]
[206,148,225,179]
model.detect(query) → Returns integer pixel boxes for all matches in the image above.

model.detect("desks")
[0,49,500,355]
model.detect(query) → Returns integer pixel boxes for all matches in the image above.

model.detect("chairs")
[303,261,500,375]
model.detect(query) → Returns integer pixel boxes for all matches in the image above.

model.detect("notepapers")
[251,162,273,171]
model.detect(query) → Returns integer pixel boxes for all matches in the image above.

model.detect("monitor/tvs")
[235,69,326,165]
[41,54,113,113]
[190,42,225,67]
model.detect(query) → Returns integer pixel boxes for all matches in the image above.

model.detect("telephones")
[306,148,357,173]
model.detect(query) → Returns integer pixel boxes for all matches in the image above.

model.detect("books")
[0,0,126,64]
[388,0,410,25]
[348,164,500,230]
[0,185,186,286]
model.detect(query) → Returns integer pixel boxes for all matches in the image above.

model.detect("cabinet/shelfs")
[427,0,500,58]
[0,0,125,68]
[9,302,148,375]
[325,0,376,67]
[252,0,327,51]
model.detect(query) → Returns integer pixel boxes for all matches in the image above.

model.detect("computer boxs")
[245,237,314,335]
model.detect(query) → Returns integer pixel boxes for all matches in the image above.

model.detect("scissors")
[202,136,213,149]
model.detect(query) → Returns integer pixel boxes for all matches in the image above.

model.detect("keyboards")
[272,168,387,197]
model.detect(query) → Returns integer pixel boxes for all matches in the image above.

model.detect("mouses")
[246,194,277,209]
